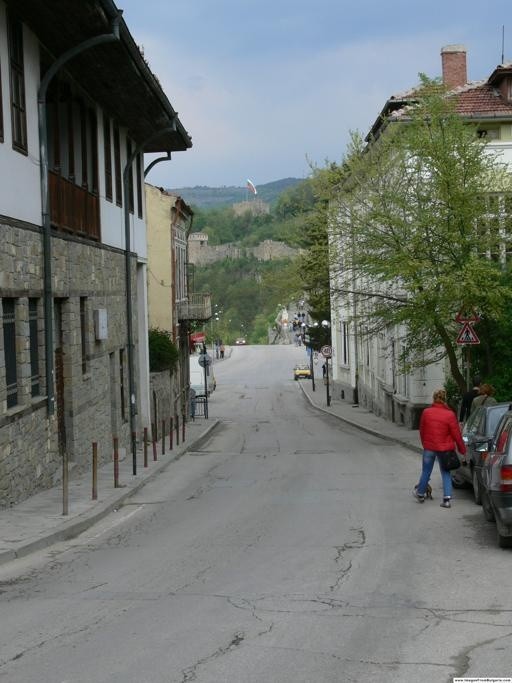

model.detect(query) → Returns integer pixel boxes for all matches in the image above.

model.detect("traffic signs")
[322,346,331,357]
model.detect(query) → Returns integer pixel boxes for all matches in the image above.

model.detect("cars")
[293,364,311,380]
[451,404,511,503]
[235,337,246,345]
[472,405,512,548]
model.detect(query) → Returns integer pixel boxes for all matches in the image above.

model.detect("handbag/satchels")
[443,452,461,470]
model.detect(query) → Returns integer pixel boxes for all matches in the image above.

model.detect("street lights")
[322,320,331,406]
[301,322,318,390]
[210,305,223,361]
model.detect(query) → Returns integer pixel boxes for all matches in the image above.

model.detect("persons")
[215,342,219,358]
[219,342,226,359]
[286,298,312,347]
[471,383,500,415]
[459,375,484,430]
[412,385,468,508]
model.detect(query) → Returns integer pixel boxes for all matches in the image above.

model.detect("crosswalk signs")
[456,323,480,345]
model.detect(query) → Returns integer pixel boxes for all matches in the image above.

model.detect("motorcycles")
[313,356,318,365]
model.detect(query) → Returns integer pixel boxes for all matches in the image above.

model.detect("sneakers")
[440,501,451,508]
[412,490,424,502]
